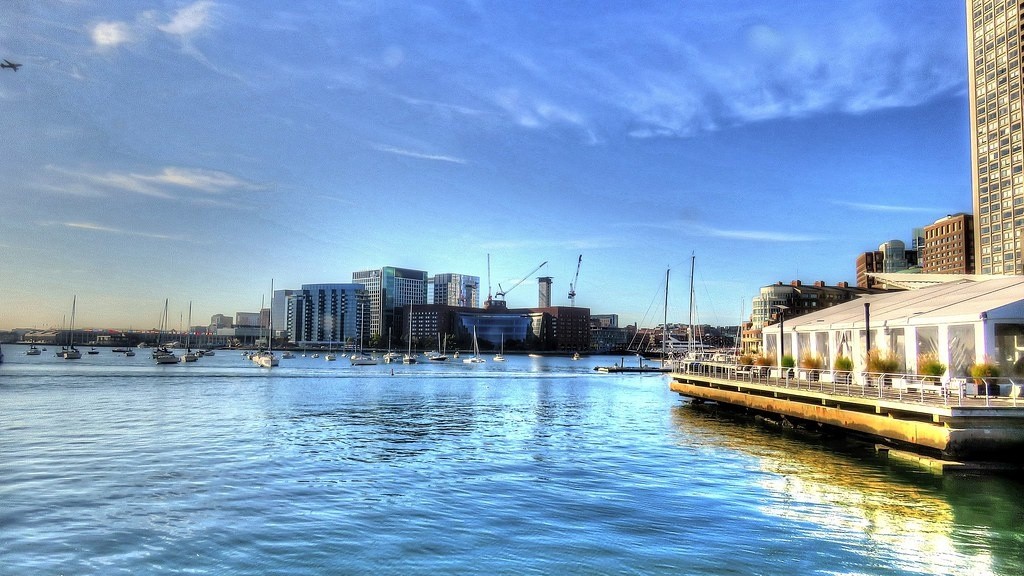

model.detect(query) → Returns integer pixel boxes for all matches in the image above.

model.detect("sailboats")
[63,296,82,360]
[157,299,179,363]
[624,268,715,360]
[179,301,199,362]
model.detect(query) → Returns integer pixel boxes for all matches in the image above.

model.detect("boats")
[27,348,41,355]
[56,351,70,357]
[205,350,215,355]
[153,348,173,358]
[238,278,507,368]
[88,351,99,355]
[126,351,135,356]
[195,351,203,357]
[112,348,129,352]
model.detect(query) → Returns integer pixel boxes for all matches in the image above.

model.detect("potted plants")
[781,354,796,381]
[740,354,755,377]
[970,356,1001,398]
[860,346,902,388]
[756,354,775,378]
[915,352,948,395]
[799,350,825,382]
[833,353,852,385]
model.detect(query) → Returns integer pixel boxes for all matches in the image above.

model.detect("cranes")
[494,261,549,300]
[568,255,582,307]
